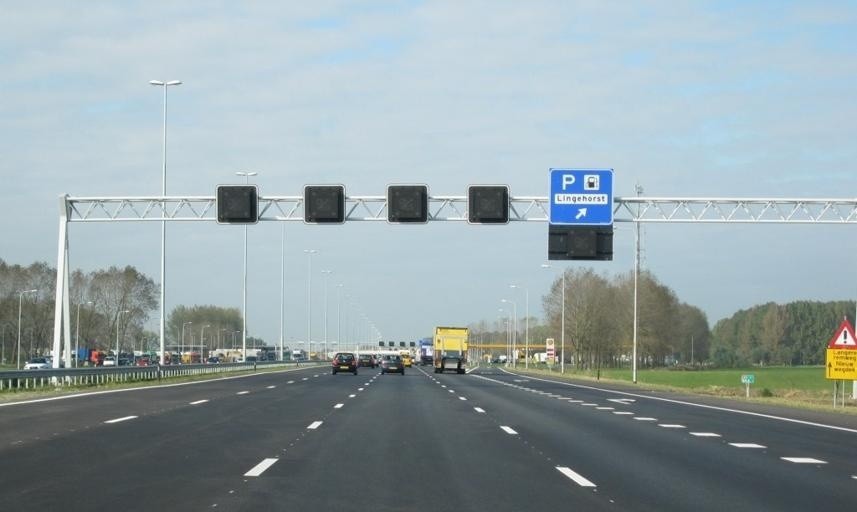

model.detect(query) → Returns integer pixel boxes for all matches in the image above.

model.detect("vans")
[333,353,357,375]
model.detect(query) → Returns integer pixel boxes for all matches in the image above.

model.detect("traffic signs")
[826,350,857,380]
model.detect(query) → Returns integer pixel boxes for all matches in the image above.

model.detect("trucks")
[292,349,306,360]
[534,353,559,363]
[433,327,470,374]
[412,339,434,367]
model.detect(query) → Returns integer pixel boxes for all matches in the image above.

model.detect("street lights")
[181,322,192,355]
[76,301,94,366]
[502,299,516,368]
[542,265,565,375]
[631,184,644,383]
[512,285,528,371]
[217,328,240,352]
[321,270,332,359]
[16,289,37,369]
[150,79,182,367]
[237,173,258,362]
[117,309,130,369]
[335,283,344,353]
[200,325,210,364]
[497,308,512,362]
[303,250,318,360]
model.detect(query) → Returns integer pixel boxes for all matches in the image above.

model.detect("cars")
[491,358,506,364]
[166,353,220,366]
[358,352,412,375]
[24,357,51,369]
[71,348,158,367]
